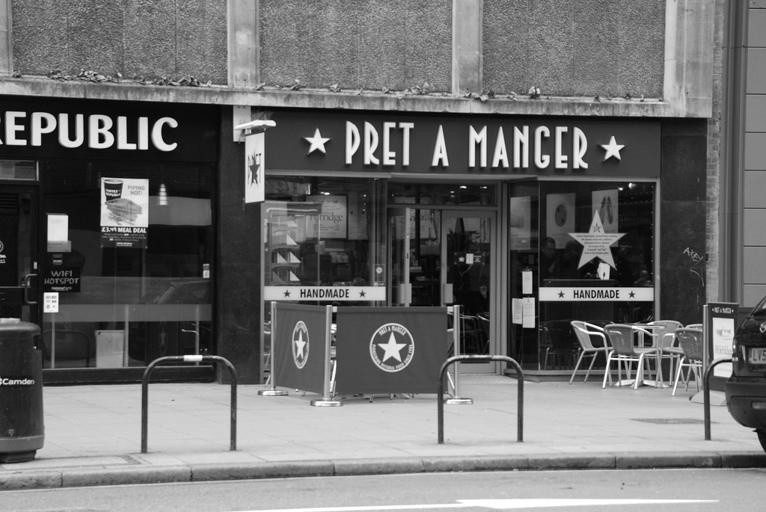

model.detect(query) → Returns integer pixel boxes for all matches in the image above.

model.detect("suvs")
[124,277,216,366]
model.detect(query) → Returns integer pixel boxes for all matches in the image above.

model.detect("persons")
[540,239,601,278]
[530,235,563,276]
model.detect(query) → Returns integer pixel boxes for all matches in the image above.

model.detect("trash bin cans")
[0,317,44,463]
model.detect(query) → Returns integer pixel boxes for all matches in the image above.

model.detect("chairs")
[570,318,703,399]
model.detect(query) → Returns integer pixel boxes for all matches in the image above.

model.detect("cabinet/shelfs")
[266,223,303,286]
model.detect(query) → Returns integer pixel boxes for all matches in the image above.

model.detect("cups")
[104,179,124,201]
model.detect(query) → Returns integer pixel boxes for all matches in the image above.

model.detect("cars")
[725,291,766,453]
